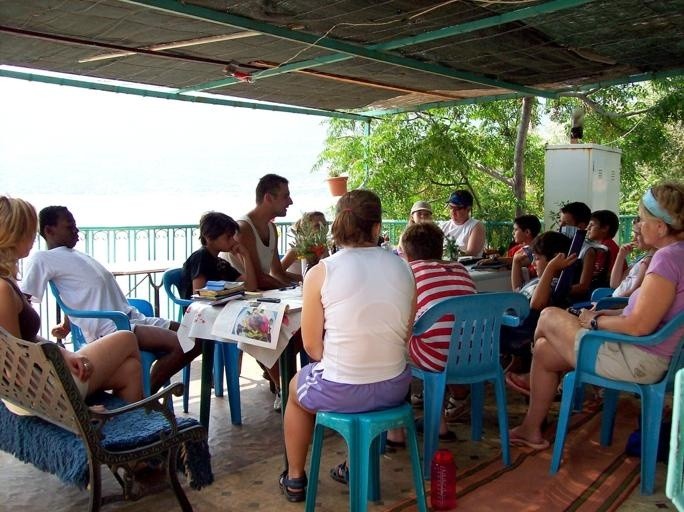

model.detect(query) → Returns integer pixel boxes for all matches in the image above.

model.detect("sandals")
[329,460,349,484]
[279,469,307,501]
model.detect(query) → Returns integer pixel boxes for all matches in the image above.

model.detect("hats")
[411,201,433,214]
[446,193,470,205]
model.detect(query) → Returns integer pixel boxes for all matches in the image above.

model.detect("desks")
[52,259,188,351]
[183,254,530,486]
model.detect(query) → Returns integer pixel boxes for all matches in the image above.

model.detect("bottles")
[450,245,460,263]
[384,238,391,252]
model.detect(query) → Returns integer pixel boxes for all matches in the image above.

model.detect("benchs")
[1,323,215,511]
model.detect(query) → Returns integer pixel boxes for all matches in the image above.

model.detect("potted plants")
[286,211,329,268]
[443,233,460,263]
[325,159,349,199]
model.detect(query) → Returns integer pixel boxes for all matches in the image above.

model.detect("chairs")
[49,276,193,415]
[162,266,254,427]
[542,308,684,496]
[587,253,655,314]
[377,288,533,480]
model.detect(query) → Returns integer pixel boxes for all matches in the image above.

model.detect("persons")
[262,211,331,394]
[19,203,200,396]
[476,201,628,402]
[386,224,477,448]
[278,191,418,502]
[0,195,144,410]
[182,175,301,410]
[505,180,682,450]
[397,189,485,258]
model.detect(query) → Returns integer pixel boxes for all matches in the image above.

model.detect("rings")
[84,363,89,367]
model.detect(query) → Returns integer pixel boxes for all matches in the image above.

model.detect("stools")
[303,403,433,512]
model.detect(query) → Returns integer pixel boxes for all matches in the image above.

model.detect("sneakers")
[385,390,471,448]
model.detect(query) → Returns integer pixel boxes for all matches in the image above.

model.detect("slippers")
[509,427,550,450]
[506,370,529,394]
